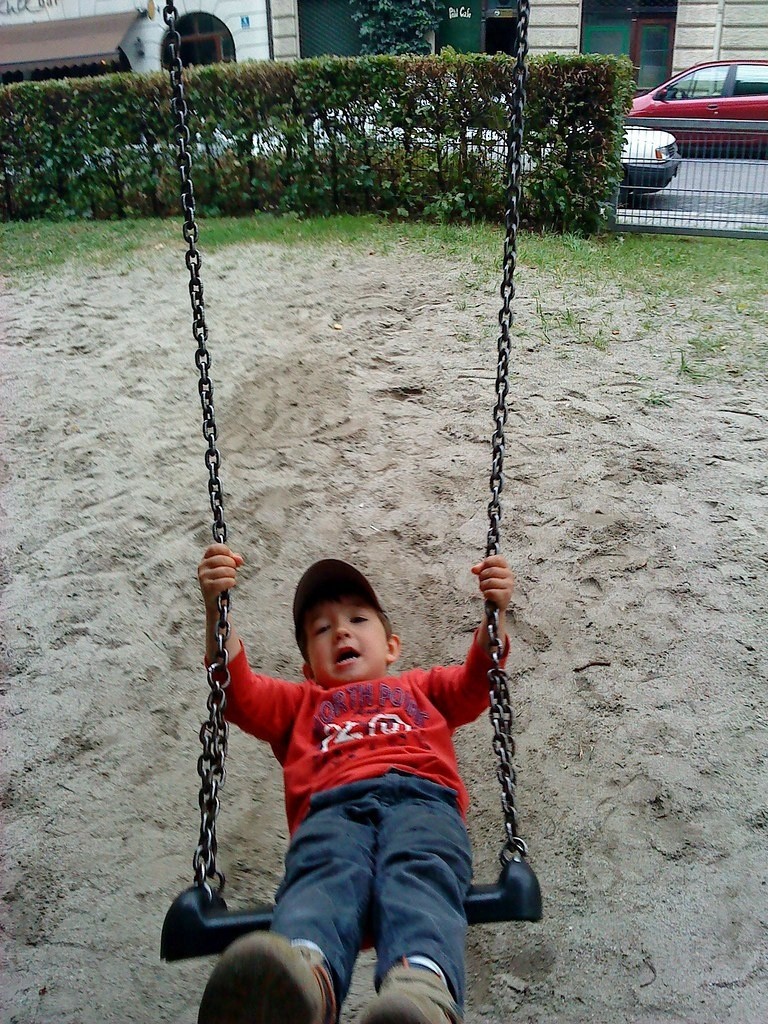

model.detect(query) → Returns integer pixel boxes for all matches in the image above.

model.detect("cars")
[626,58,768,153]
[251,80,680,207]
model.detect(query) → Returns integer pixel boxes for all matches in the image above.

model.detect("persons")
[197,543,513,1024]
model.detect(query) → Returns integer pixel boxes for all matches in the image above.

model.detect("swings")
[157,0,544,965]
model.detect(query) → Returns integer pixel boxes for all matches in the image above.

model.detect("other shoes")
[198,931,337,1024]
[356,961,464,1024]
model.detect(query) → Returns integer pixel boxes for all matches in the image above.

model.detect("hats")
[292,559,388,660]
